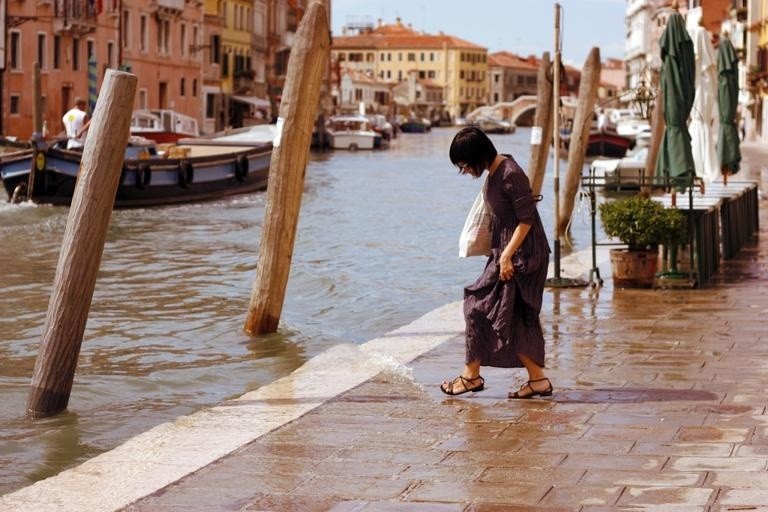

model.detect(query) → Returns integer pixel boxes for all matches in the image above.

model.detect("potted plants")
[599,193,692,289]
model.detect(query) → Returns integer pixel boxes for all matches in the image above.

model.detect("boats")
[399,120,425,134]
[559,128,635,159]
[0,124,278,211]
[324,115,382,151]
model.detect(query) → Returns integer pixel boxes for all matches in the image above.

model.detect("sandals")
[508,375,554,400]
[439,374,486,396]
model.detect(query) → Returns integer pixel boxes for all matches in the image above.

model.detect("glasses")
[462,163,471,170]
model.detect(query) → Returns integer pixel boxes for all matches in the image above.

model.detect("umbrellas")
[651,0,698,207]
[690,26,717,195]
[717,31,742,187]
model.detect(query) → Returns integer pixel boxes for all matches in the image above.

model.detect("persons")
[438,125,554,399]
[76,119,90,140]
[62,97,89,152]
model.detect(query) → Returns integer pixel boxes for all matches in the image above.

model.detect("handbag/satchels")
[456,173,496,261]
